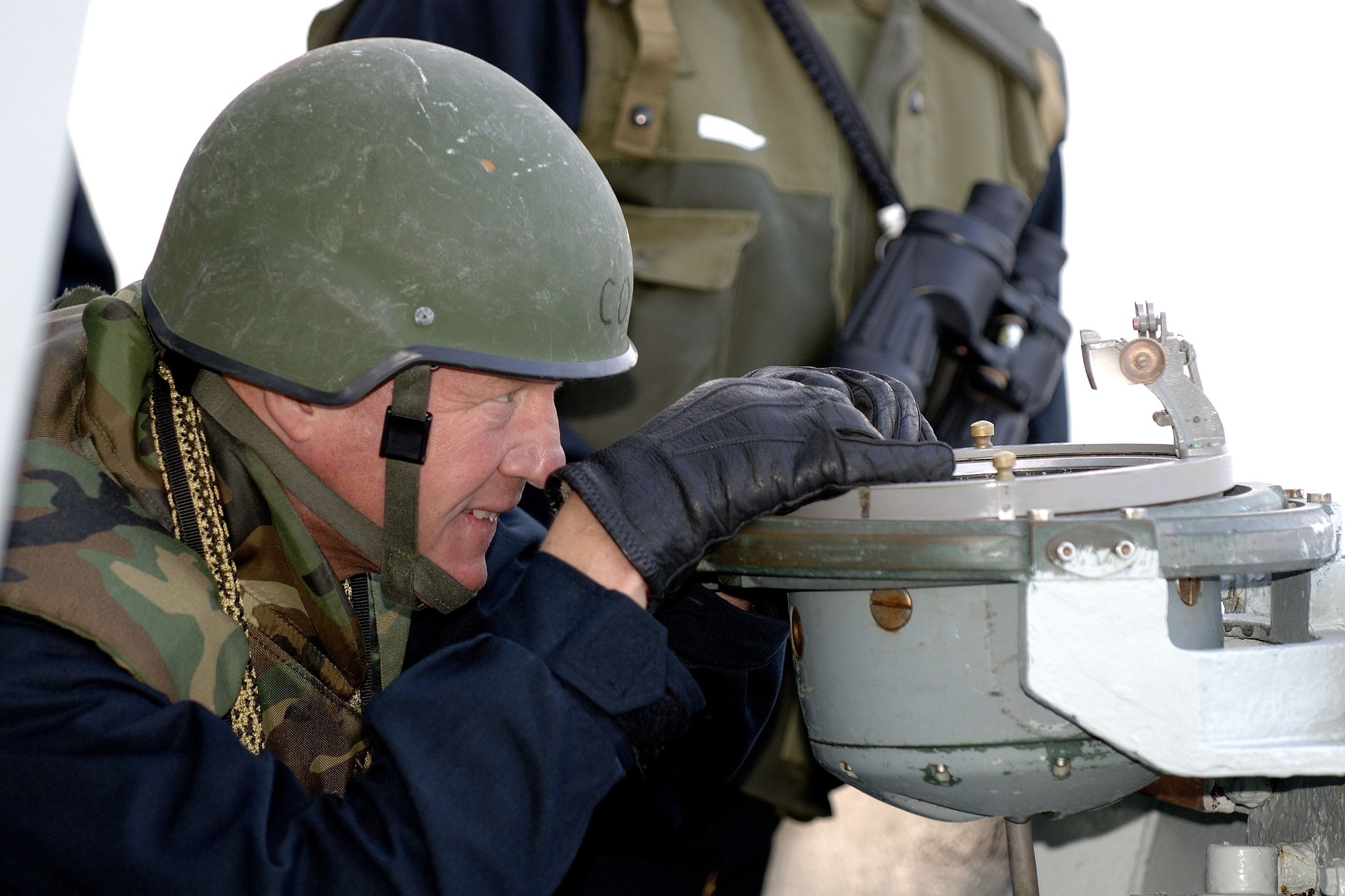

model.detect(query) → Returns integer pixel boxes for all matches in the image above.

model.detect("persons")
[0,32,960,896]
[296,0,1069,896]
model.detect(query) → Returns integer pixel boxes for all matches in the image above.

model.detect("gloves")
[740,365,938,443]
[543,377,957,597]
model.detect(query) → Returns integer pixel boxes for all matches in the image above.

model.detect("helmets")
[142,39,639,409]
